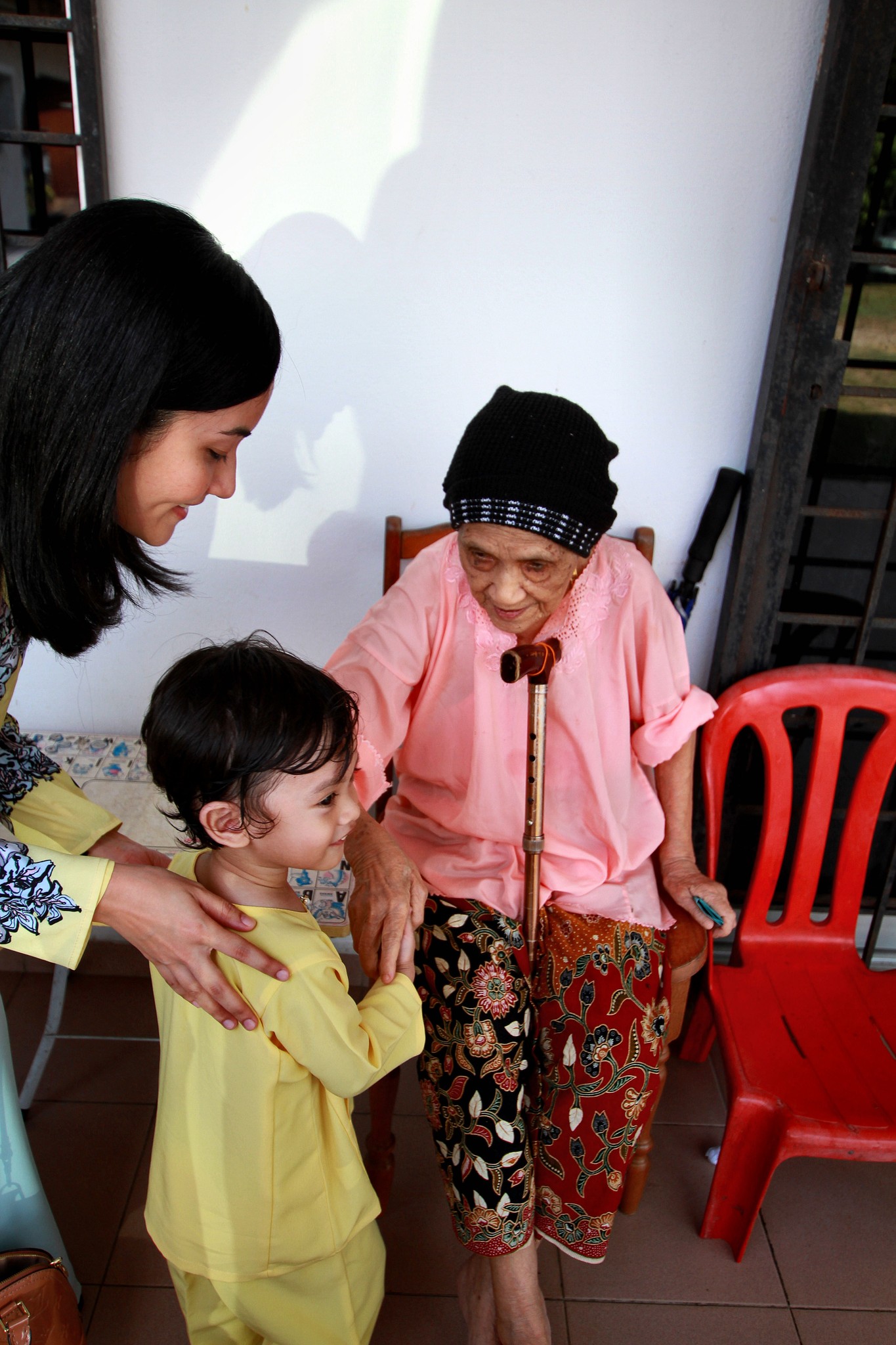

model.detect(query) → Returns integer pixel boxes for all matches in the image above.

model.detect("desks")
[0,732,367,1121]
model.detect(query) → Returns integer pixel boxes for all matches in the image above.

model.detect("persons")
[0,200,291,1303]
[143,631,426,1345]
[323,386,738,1345]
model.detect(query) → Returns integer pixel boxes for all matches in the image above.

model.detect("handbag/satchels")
[0,1247,86,1345]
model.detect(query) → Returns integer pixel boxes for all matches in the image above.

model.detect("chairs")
[364,517,709,1215]
[680,663,896,1264]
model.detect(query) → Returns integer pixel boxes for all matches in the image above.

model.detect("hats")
[441,385,619,559]
